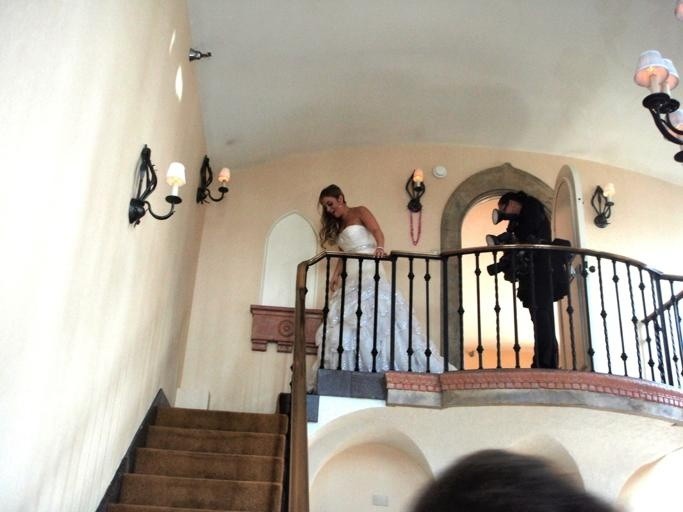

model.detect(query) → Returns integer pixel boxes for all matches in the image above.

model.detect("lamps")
[633,49,683,162]
[590,181,616,230]
[130,138,185,224]
[198,151,230,205]
[404,167,424,243]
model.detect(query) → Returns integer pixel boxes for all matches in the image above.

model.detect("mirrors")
[260,210,319,310]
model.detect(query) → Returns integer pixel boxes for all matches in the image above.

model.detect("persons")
[485,193,559,368]
[314,184,457,373]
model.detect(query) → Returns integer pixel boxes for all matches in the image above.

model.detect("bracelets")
[376,245,386,250]
